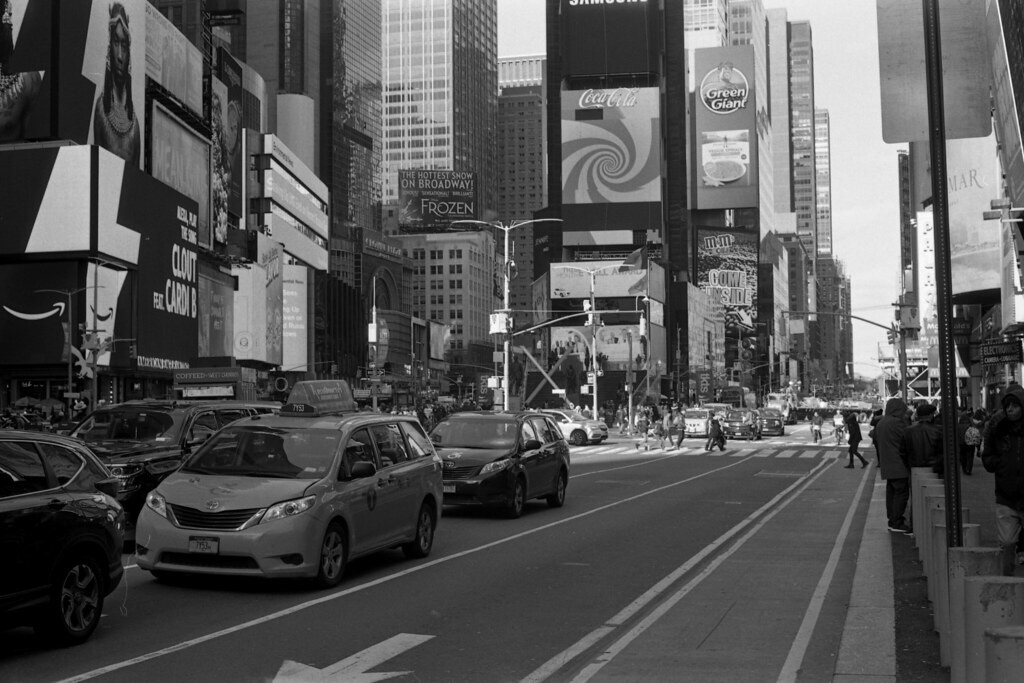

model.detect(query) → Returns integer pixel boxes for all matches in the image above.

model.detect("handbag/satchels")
[869,429,875,438]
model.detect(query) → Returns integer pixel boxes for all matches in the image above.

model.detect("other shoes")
[876,464,881,468]
[861,462,869,468]
[1017,551,1024,564]
[844,464,854,468]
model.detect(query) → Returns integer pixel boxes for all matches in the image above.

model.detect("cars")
[524,407,609,447]
[427,408,572,519]
[134,379,447,594]
[677,399,785,441]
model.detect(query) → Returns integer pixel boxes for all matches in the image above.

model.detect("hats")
[917,405,936,415]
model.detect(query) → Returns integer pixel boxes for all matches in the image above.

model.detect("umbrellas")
[12,396,66,419]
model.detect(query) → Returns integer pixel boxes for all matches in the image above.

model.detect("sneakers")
[888,520,909,532]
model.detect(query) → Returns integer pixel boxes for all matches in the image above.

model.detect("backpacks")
[965,425,981,446]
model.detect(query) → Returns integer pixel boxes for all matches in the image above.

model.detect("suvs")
[66,397,258,558]
[0,429,125,646]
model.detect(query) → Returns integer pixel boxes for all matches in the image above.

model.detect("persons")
[573,404,628,435]
[0,400,106,429]
[811,410,845,443]
[869,397,986,533]
[364,392,484,432]
[634,404,728,452]
[845,415,869,469]
[982,383,1024,577]
[86,4,141,170]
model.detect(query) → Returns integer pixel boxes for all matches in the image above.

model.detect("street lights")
[553,264,635,420]
[453,216,563,408]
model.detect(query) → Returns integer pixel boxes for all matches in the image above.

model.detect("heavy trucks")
[765,392,872,425]
